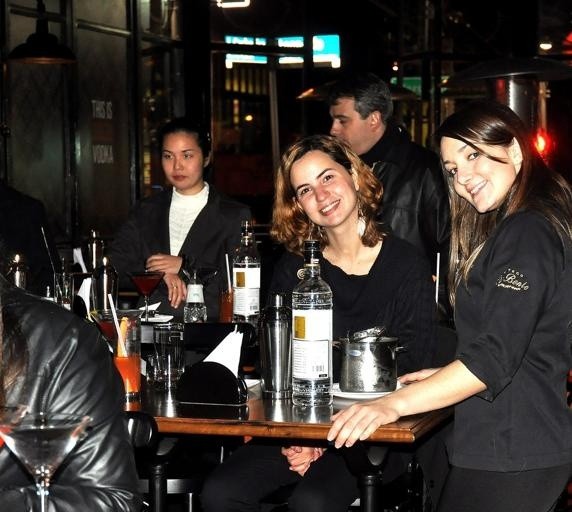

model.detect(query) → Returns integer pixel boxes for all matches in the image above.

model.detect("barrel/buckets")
[339,324,420,394]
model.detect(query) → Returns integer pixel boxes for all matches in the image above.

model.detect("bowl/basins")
[102,309,145,327]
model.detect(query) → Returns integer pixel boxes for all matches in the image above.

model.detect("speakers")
[451,0,572,82]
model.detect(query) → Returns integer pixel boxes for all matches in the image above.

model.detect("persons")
[0,271,141,512]
[200,72,456,512]
[0,182,51,294]
[107,117,243,324]
[328,95,571,511]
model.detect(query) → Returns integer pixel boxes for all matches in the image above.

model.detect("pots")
[333,337,408,393]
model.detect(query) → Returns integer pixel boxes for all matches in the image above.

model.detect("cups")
[152,324,186,387]
[113,341,144,398]
[221,291,236,324]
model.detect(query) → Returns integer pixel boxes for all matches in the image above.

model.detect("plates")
[330,384,404,401]
[141,313,175,323]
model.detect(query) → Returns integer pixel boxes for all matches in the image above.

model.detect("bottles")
[184,267,209,324]
[258,241,333,407]
[54,272,74,312]
[14,264,26,290]
[234,221,267,321]
[91,257,120,311]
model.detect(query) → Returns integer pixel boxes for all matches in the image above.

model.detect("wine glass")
[0,413,92,511]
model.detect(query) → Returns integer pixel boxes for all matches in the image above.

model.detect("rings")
[172,284,178,290]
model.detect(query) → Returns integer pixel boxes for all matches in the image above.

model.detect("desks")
[121,381,456,510]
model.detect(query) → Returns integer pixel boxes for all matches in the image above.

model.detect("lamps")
[8,0,79,66]
